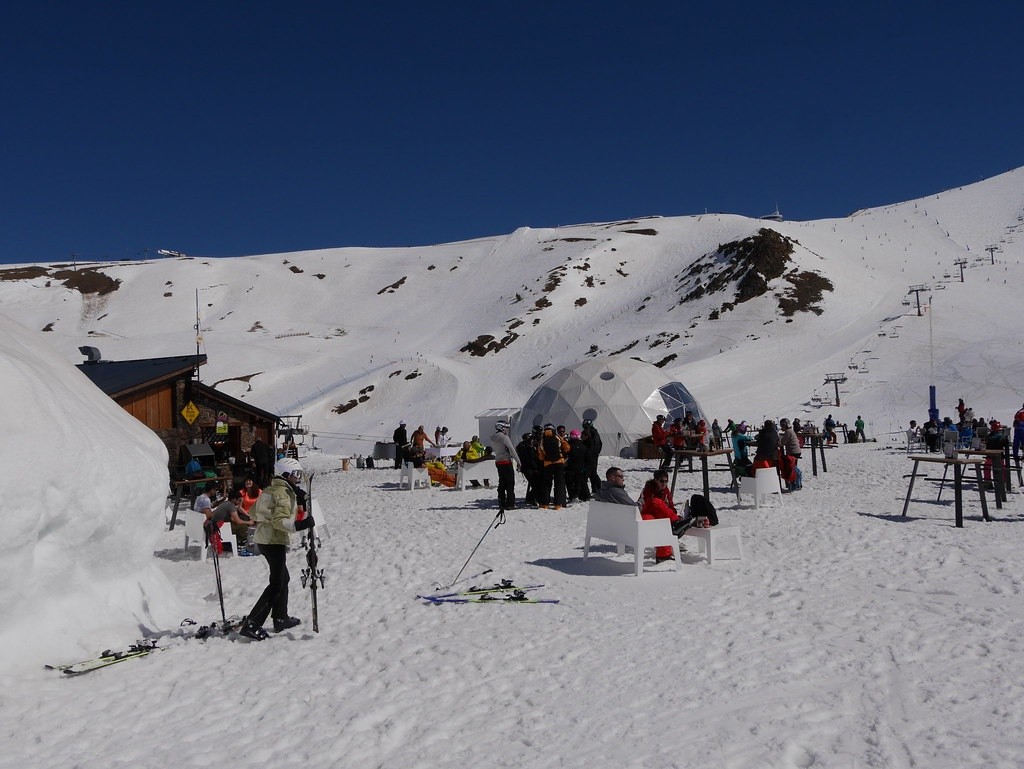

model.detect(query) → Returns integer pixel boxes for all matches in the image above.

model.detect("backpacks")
[690,494,719,526]
[784,466,803,491]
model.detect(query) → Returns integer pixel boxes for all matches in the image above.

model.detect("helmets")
[531,426,543,434]
[522,432,533,443]
[274,457,303,477]
[495,420,511,431]
[657,410,706,427]
[780,418,791,431]
[544,423,556,432]
[581,419,593,428]
[569,430,581,439]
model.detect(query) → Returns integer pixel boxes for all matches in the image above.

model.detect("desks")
[670,449,742,506]
[169,476,231,531]
[679,526,745,565]
[780,432,827,475]
[833,425,849,443]
[901,456,989,528]
[424,448,463,460]
[937,449,1008,508]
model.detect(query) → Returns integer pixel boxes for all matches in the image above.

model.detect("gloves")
[294,516,315,531]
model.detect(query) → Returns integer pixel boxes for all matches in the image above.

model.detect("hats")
[399,419,406,425]
[737,421,748,434]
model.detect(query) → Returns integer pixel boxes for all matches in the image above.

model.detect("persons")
[239,458,316,640]
[822,414,839,445]
[651,411,819,491]
[437,426,452,445]
[855,415,866,443]
[489,418,603,510]
[194,474,306,557]
[393,419,409,469]
[593,467,697,563]
[454,436,496,487]
[409,424,434,467]
[906,398,1024,488]
[251,436,270,489]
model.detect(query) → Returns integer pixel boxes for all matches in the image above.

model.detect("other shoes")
[500,495,592,510]
[656,555,675,563]
[679,541,687,552]
[238,549,255,557]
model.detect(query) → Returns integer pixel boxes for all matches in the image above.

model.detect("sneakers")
[273,616,300,634]
[239,620,269,641]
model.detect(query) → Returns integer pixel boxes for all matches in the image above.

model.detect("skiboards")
[45,614,247,674]
[301,472,326,634]
[416,584,560,604]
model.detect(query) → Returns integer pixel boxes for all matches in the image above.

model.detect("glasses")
[559,430,565,433]
[293,471,301,479]
[613,474,623,479]
[657,479,668,483]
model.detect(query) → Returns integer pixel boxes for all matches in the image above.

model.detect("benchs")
[456,460,500,491]
[184,508,238,562]
[583,500,682,575]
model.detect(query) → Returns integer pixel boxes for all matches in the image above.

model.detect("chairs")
[400,458,421,488]
[408,462,431,492]
[739,467,784,509]
[904,425,1013,459]
